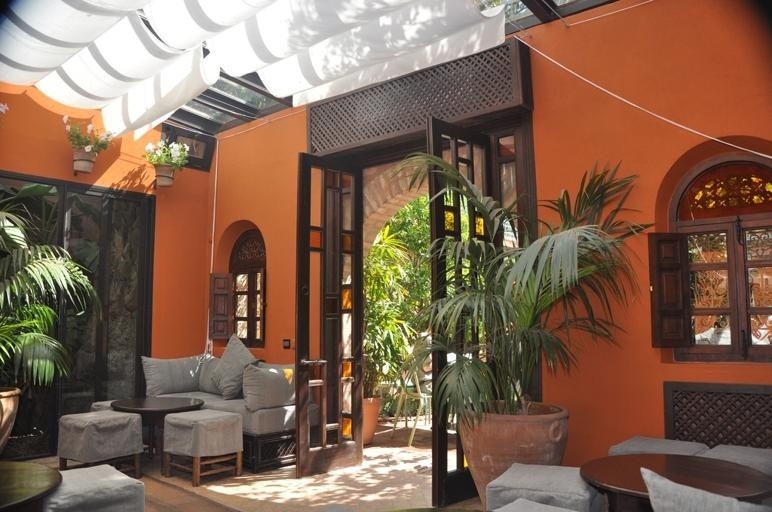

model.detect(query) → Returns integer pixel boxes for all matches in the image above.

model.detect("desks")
[581,453,771,510]
[112,397,205,460]
[0,459,63,512]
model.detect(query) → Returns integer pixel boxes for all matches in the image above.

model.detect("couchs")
[155,387,300,470]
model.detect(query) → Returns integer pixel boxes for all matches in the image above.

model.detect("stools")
[90,399,119,410]
[162,408,246,488]
[485,498,577,512]
[56,411,146,481]
[42,465,148,510]
[481,456,606,511]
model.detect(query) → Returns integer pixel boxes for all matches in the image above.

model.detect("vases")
[153,162,174,186]
[71,151,94,174]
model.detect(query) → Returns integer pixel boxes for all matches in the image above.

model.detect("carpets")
[11,450,244,512]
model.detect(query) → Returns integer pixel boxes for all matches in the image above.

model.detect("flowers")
[0,103,8,115]
[61,112,114,158]
[142,137,192,170]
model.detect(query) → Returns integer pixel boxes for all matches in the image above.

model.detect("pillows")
[640,466,772,512]
[700,442,771,474]
[198,352,229,395]
[240,361,303,412]
[212,334,265,397]
[605,432,709,462]
[139,355,206,401]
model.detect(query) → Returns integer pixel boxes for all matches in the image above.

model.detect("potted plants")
[386,149,656,506]
[1,208,103,450]
[363,220,424,447]
